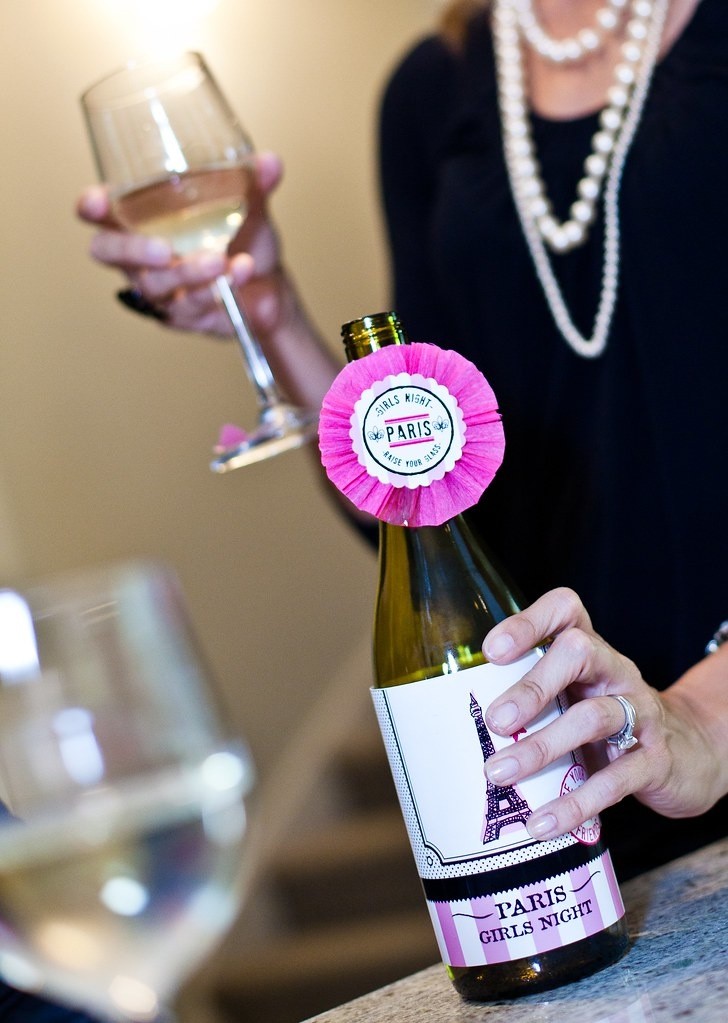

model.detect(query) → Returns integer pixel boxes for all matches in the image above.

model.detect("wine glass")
[0,564,248,1022]
[79,42,332,474]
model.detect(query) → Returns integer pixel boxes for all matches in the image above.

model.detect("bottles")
[336,312,629,1001]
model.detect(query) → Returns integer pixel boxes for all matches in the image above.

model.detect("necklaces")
[493,0,675,359]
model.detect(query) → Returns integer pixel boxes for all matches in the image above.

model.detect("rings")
[604,693,638,751]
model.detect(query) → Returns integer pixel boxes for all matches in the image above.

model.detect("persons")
[78,0,728,886]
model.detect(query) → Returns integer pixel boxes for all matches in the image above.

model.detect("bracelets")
[706,622,728,653]
